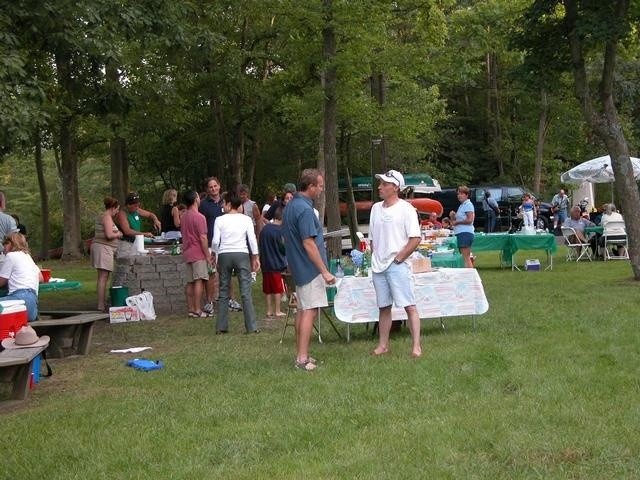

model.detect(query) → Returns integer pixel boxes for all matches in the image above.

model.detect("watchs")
[394,257,402,264]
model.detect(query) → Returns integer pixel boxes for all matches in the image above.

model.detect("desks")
[330,232,554,343]
[584,226,603,259]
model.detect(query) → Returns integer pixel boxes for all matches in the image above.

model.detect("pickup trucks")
[432,185,553,230]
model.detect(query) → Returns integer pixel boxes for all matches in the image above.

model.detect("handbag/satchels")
[126,291,157,320]
[206,262,220,302]
[494,208,499,217]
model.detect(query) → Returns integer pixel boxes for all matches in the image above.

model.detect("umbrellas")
[560,153,640,204]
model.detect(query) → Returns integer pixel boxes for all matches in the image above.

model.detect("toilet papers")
[130,234,145,255]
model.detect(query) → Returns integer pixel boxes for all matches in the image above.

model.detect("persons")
[482,192,501,233]
[91,176,293,335]
[368,169,423,359]
[0,188,44,384]
[285,168,334,370]
[449,185,475,269]
[415,206,453,231]
[513,191,627,259]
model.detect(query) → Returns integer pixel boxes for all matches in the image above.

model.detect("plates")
[138,247,169,256]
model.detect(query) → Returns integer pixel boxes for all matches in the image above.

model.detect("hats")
[375,170,405,191]
[1,325,50,349]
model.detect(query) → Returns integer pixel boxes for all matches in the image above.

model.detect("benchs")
[0,311,110,400]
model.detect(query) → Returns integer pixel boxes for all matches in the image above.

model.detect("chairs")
[602,221,630,261]
[561,236,591,261]
[560,227,593,263]
[280,273,341,344]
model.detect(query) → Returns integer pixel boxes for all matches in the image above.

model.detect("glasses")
[128,195,139,200]
[386,171,401,184]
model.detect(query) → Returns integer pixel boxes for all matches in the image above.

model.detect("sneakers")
[203,302,214,314]
[228,299,243,313]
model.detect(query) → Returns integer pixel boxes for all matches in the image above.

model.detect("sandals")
[295,360,317,371]
[188,310,194,317]
[193,312,214,318]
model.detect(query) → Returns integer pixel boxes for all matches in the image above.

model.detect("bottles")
[338,251,368,278]
[171,236,181,255]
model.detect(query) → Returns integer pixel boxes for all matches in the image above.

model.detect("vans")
[323,173,441,226]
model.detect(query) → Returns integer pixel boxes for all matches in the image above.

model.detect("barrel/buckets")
[109,285,130,307]
[40,268,52,282]
[1,312,28,342]
